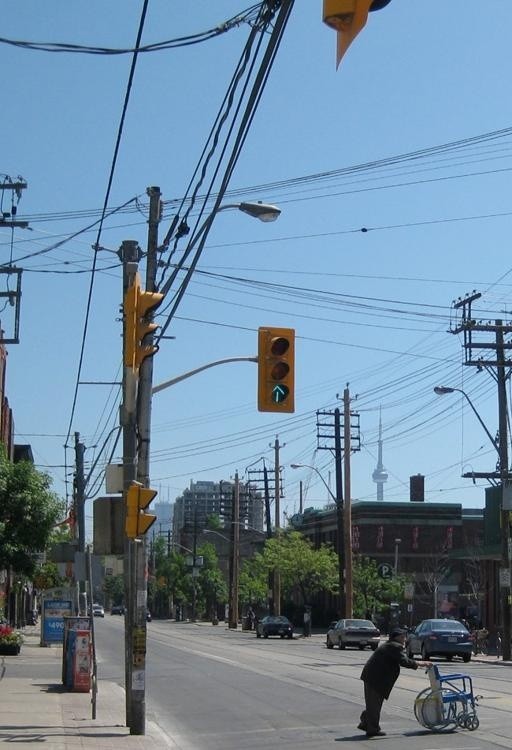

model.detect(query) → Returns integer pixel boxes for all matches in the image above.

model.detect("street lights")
[202,469,240,626]
[434,319,511,662]
[114,200,281,733]
[165,501,197,623]
[219,438,281,614]
[290,408,344,622]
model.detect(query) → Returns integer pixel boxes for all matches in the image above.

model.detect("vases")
[0,644,20,656]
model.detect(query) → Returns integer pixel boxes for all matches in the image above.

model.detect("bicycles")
[473,627,502,659]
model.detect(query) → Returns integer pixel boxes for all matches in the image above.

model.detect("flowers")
[0,627,24,648]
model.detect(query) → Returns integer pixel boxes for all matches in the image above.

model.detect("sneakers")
[357,723,387,736]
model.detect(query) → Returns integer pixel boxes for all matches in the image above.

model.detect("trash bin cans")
[242,616,252,630]
[63,614,91,686]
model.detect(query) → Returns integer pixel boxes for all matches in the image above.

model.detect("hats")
[389,627,408,638]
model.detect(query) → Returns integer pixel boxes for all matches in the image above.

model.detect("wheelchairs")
[413,665,482,732]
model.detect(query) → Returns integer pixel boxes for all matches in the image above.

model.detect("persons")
[246,605,256,623]
[303,608,312,638]
[362,607,501,654]
[355,628,434,736]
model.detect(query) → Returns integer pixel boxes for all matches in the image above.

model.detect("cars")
[90,604,105,618]
[326,618,380,651]
[255,614,294,639]
[405,618,472,662]
[112,605,124,616]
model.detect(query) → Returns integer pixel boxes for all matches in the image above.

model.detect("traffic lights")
[123,484,159,538]
[256,326,295,413]
[123,276,164,365]
